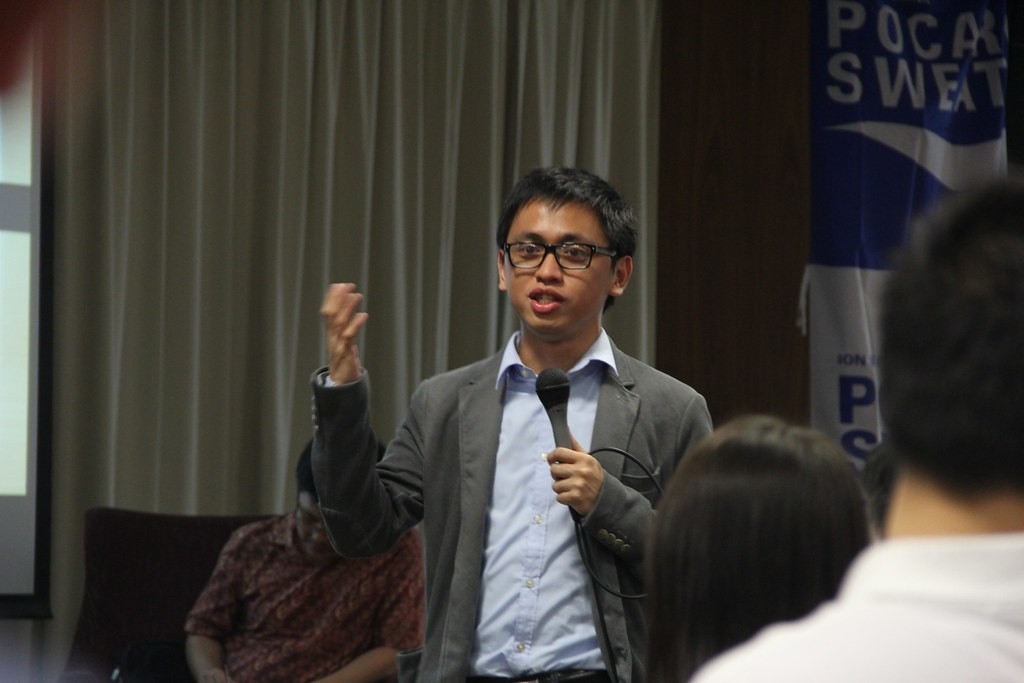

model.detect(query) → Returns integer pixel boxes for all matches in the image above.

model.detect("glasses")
[294,504,325,534]
[504,242,616,270]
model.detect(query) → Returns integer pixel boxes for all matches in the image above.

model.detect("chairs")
[61,507,284,683]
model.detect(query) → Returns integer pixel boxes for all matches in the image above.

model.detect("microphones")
[536,366,580,523]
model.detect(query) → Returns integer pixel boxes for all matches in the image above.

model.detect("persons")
[310,166,713,683]
[691,172,1024,683]
[183,437,428,683]
[643,413,865,683]
